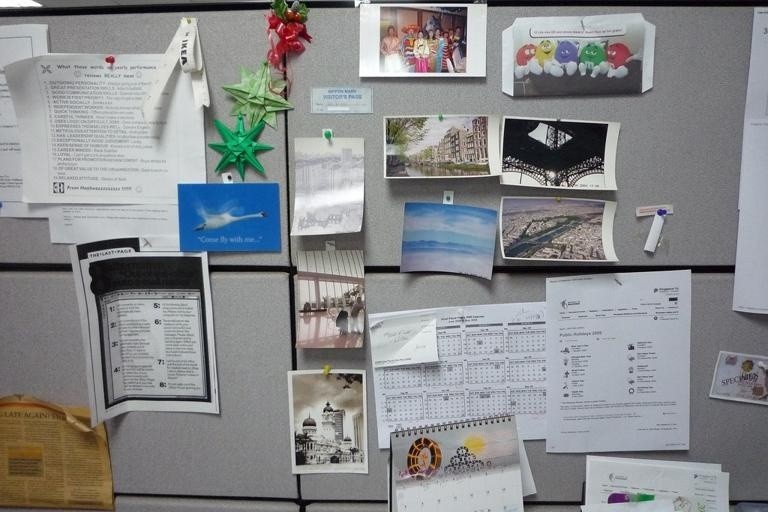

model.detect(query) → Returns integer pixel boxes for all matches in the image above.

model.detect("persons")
[380,26,401,56]
[400,24,420,72]
[413,30,429,72]
[433,27,463,72]
[427,30,439,71]
[351,296,364,334]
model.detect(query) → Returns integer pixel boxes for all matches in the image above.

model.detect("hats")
[401,24,420,33]
